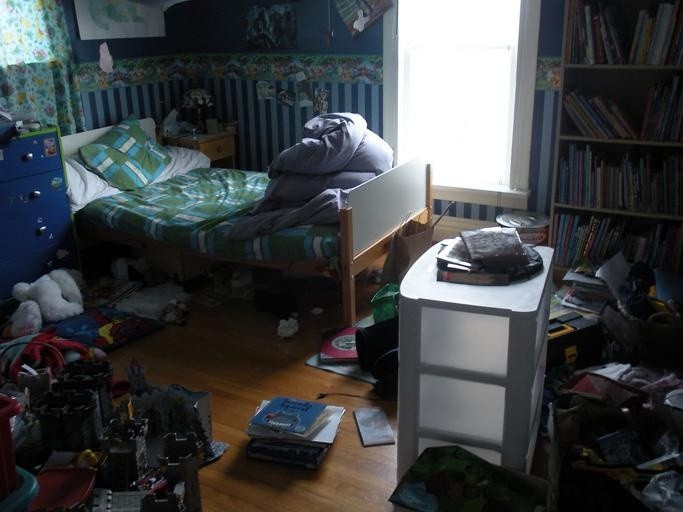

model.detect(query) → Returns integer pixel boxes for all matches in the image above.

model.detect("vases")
[192,111,206,133]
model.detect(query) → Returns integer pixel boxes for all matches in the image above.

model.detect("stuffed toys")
[11,268,95,338]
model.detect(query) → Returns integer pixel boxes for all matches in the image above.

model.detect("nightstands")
[163,132,236,170]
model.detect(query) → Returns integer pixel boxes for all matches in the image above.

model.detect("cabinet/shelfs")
[547,1,682,281]
[397,237,555,488]
[0,123,83,313]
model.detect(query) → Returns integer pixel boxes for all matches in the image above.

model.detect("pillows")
[77,115,170,191]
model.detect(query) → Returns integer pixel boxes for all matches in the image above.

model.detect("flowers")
[182,88,216,115]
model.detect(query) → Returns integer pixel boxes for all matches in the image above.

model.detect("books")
[244,397,349,470]
[553,2,680,313]
[318,325,363,362]
[354,407,395,446]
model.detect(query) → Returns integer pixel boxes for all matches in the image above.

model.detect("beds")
[60,117,433,328]
